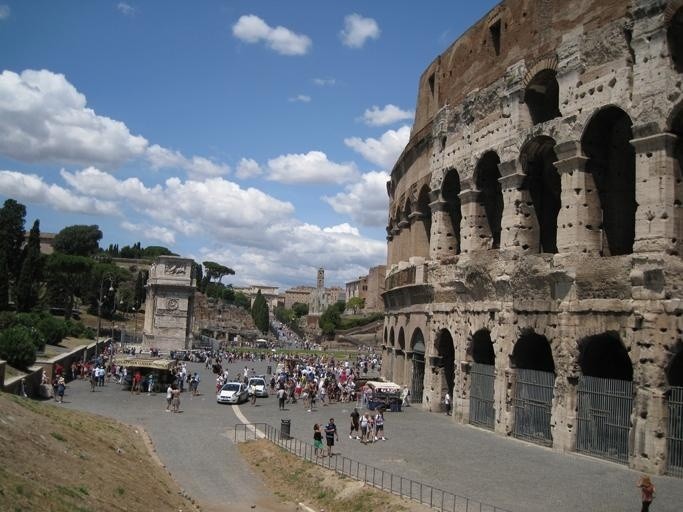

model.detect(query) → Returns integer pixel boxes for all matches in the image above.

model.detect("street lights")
[95,272,136,369]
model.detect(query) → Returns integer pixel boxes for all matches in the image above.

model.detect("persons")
[349,407,386,444]
[42,343,120,404]
[445,391,452,415]
[314,418,339,456]
[637,474,656,512]
[115,341,409,413]
[19,378,29,398]
[274,324,317,351]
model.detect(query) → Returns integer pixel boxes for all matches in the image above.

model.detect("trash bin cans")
[280,418,291,439]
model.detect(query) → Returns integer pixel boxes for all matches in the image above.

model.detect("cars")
[217,382,249,404]
[248,377,267,397]
